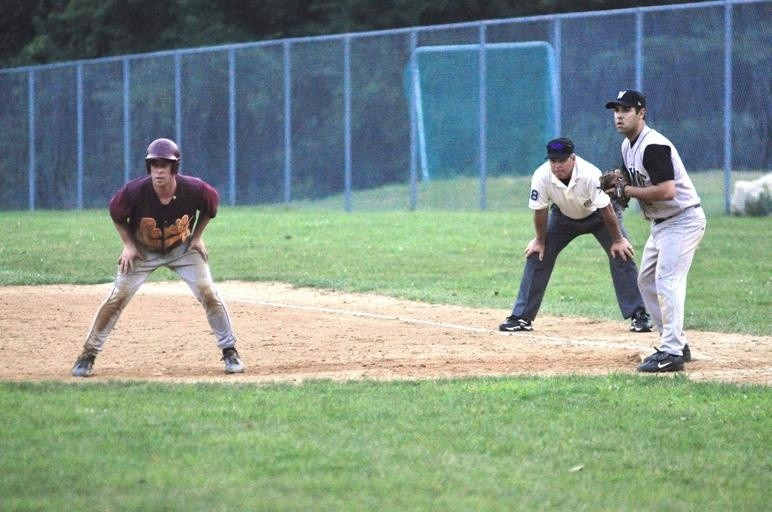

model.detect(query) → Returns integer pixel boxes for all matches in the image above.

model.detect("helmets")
[144,139,180,174]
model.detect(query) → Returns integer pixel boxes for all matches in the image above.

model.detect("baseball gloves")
[601,172,629,208]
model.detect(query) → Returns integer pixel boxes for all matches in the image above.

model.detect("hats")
[544,138,574,160]
[604,88,645,109]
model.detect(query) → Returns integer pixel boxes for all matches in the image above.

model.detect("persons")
[598,89,707,374]
[499,138,654,333]
[71,140,244,376]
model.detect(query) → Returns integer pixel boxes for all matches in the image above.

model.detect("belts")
[654,202,701,225]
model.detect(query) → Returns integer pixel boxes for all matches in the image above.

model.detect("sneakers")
[630,311,652,332]
[223,347,244,372]
[645,343,691,364]
[71,354,95,377]
[638,351,685,372]
[499,315,534,331]
